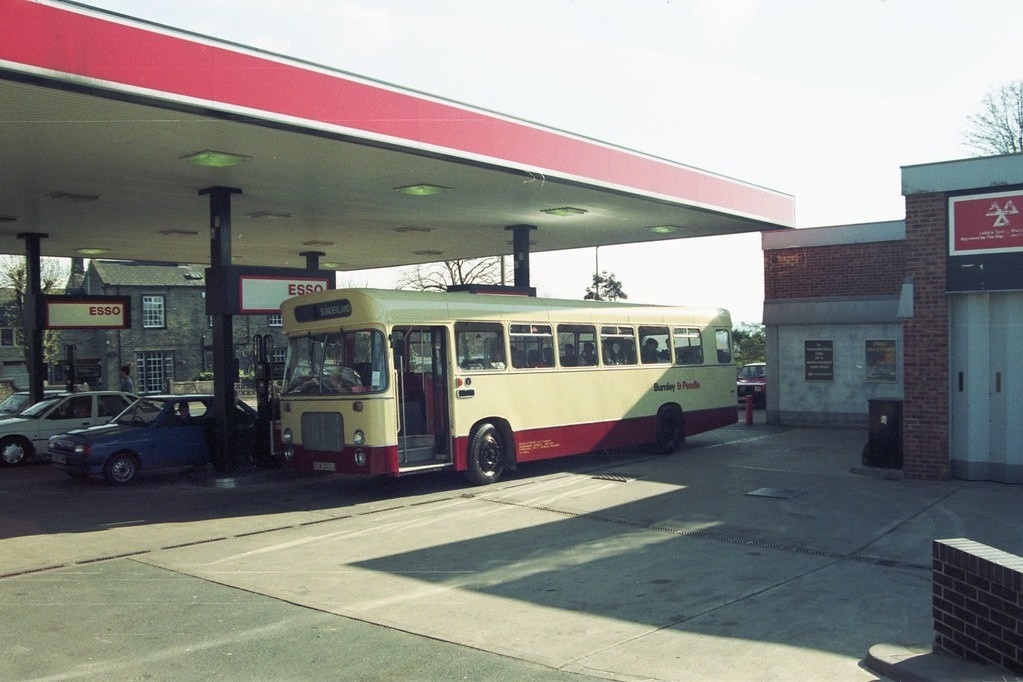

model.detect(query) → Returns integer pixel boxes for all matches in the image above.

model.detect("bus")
[280,285,741,485]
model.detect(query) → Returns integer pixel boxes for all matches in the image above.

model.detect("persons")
[502,334,683,367]
[117,366,133,393]
[175,401,194,426]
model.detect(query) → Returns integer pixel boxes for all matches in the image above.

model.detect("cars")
[0,392,66,421]
[47,393,263,485]
[737,362,767,401]
[0,391,164,469]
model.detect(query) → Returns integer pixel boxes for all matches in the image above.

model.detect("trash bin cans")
[862,399,903,469]
